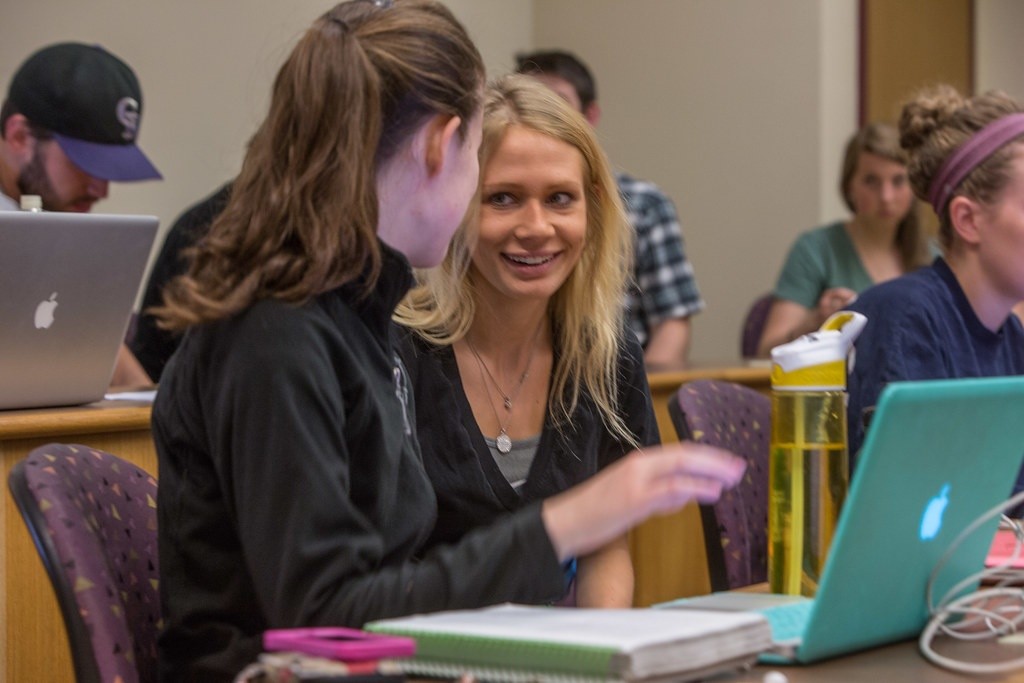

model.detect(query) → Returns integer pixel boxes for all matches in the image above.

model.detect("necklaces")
[467,320,544,408]
[464,336,512,453]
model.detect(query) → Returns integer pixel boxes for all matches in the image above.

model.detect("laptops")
[0,210,160,410]
[653,375,1024,666]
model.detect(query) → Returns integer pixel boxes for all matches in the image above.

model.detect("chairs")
[668,376,825,595]
[10,440,160,683]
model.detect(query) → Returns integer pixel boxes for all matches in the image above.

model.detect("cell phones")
[263,625,415,662]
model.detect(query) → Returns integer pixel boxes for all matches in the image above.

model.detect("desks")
[0,395,162,683]
[692,581,1023,683]
[625,365,774,608]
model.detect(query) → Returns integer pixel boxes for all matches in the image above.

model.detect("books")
[364,600,772,683]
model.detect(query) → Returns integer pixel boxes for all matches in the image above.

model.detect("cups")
[767,310,869,598]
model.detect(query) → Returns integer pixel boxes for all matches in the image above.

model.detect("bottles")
[19,194,46,213]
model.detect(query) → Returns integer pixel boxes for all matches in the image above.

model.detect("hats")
[10,42,164,181]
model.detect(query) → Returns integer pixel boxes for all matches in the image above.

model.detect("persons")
[155,0,746,683]
[132,174,237,384]
[756,124,942,358]
[513,50,704,371]
[844,85,1024,512]
[393,71,662,610]
[0,44,163,394]
